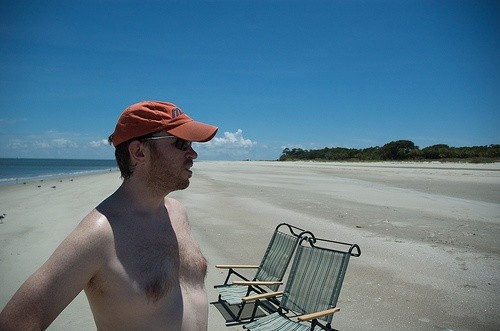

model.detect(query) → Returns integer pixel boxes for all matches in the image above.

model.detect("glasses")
[139,136,192,151]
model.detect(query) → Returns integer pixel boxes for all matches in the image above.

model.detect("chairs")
[242,236,362,331]
[208,222,316,325]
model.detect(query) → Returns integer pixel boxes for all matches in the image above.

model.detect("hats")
[112,101,219,147]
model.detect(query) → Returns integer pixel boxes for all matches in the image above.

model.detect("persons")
[0,100,219,331]
